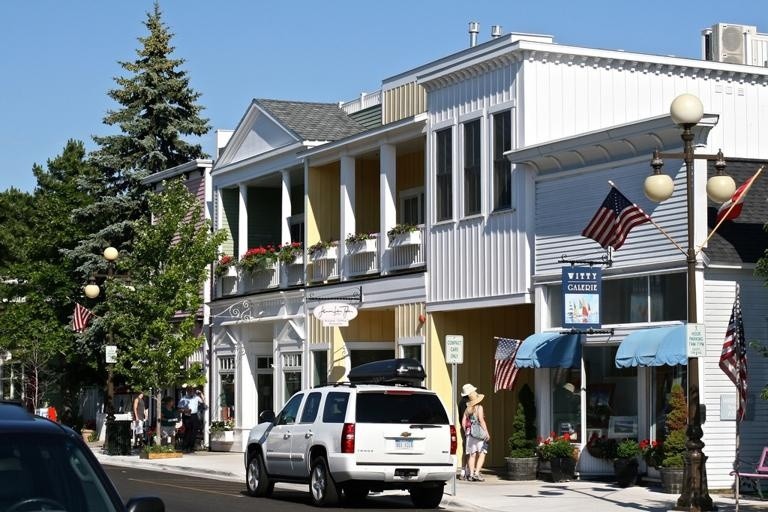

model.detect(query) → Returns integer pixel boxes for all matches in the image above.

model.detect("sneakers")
[460,473,484,481]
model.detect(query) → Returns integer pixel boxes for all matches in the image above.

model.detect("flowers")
[535,431,579,462]
[214,240,302,278]
[639,439,662,466]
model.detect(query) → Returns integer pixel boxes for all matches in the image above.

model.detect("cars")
[0,398,169,511]
[549,384,690,447]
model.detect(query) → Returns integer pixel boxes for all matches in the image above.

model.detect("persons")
[461,390,491,482]
[132,387,206,454]
[457,383,479,481]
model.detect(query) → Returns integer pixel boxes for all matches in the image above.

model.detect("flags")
[582,186,650,252]
[716,176,754,221]
[493,337,522,393]
[715,284,754,426]
[72,301,90,334]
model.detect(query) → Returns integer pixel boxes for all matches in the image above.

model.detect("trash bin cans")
[105,420,133,455]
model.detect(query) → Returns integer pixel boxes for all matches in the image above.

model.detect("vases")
[647,464,661,478]
[550,457,575,483]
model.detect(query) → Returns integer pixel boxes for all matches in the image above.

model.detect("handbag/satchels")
[471,420,487,439]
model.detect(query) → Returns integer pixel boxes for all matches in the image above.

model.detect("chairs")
[728,447,767,512]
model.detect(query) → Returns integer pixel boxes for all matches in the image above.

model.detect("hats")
[466,392,484,406]
[461,384,477,397]
[562,383,575,393]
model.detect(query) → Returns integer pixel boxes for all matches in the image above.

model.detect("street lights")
[84,241,136,455]
[644,93,736,511]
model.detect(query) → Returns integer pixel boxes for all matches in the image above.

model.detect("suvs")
[243,358,458,510]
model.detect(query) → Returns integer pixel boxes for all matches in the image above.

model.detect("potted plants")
[503,384,540,481]
[613,439,641,488]
[209,421,234,443]
[657,383,689,495]
[307,223,422,261]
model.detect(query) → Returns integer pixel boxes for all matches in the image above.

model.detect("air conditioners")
[711,21,757,64]
